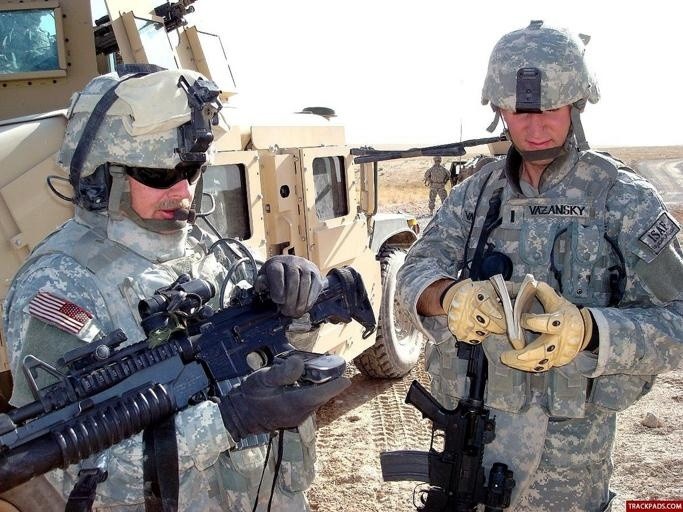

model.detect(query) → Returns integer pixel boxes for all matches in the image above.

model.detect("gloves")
[255,253,323,316]
[441,277,517,346]
[217,357,352,444]
[501,281,594,375]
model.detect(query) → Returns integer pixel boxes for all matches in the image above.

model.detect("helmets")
[480,24,601,111]
[432,155,443,162]
[54,65,223,177]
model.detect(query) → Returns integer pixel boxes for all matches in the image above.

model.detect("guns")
[380,343,516,512]
[0,265,376,494]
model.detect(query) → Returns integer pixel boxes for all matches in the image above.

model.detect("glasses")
[115,164,206,190]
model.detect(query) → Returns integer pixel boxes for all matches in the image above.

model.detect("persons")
[423,155,448,216]
[0,66,353,511]
[392,20,683,512]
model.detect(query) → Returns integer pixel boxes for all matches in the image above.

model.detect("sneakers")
[429,209,434,216]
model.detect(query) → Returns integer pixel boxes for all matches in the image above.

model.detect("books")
[489,271,537,349]
